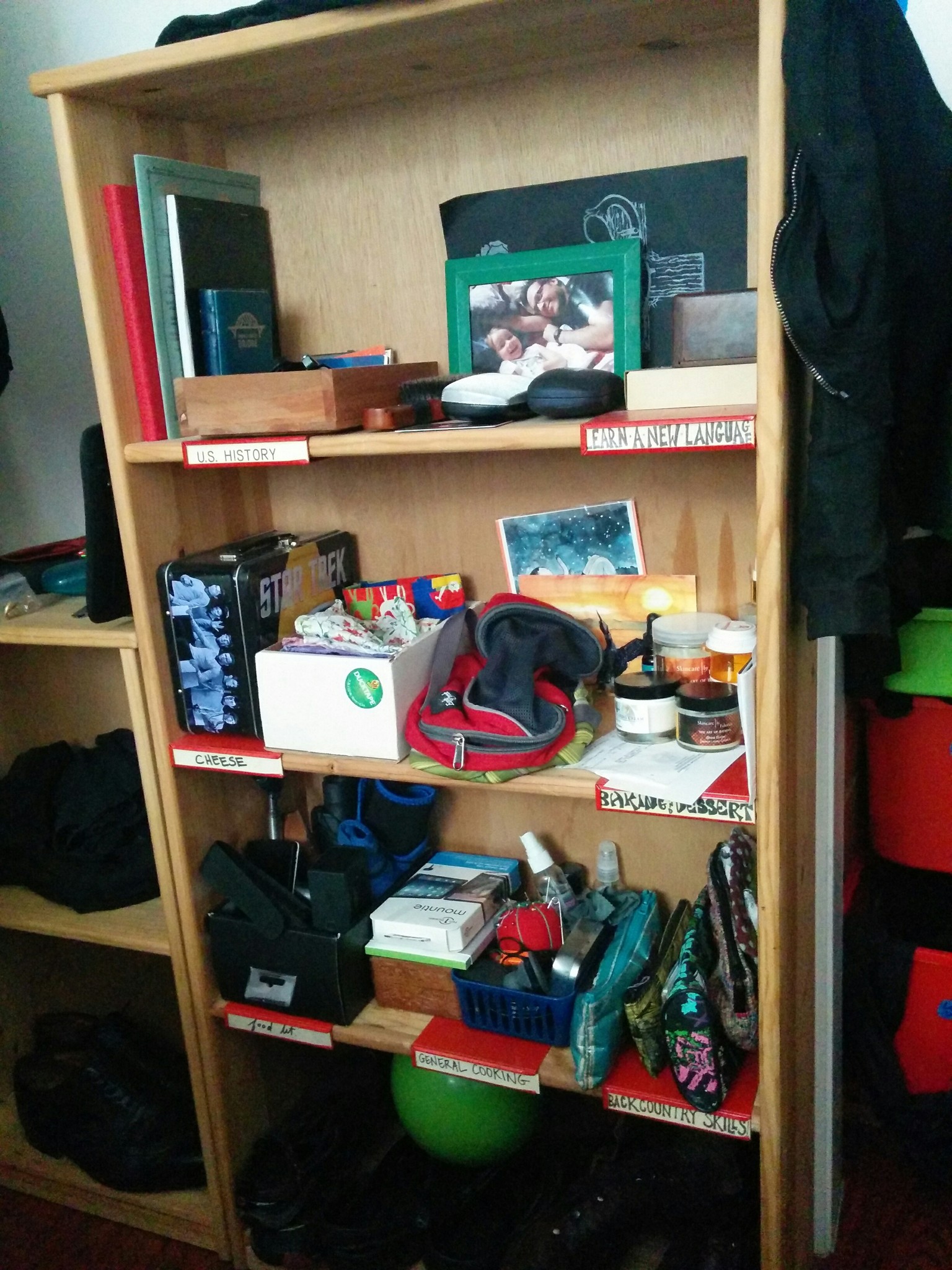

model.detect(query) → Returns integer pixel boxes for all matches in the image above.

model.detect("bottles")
[613,611,742,750]
[518,828,574,900]
[592,841,628,894]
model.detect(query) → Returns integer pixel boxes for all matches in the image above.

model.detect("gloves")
[361,779,437,901]
[311,776,395,899]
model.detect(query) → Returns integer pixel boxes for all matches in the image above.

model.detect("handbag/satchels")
[404,592,603,770]
[343,573,464,621]
[0,726,160,915]
[10,1008,206,1193]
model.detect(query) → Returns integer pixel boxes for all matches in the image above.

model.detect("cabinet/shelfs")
[27,0,854,1270]
[0,597,228,1259]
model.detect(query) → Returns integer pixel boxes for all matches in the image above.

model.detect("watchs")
[553,329,563,345]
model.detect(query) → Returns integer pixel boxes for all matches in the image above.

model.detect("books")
[622,364,758,411]
[101,151,283,444]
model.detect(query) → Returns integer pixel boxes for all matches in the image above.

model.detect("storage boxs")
[444,235,642,380]
[372,955,462,1020]
[451,967,577,1050]
[370,851,522,955]
[207,898,373,1028]
[156,528,360,734]
[623,361,758,411]
[173,362,439,438]
[254,601,488,759]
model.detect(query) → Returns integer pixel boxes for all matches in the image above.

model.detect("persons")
[470,272,616,385]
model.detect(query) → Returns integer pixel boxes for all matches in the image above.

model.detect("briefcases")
[157,530,361,740]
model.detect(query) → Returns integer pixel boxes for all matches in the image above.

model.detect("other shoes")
[234,1090,761,1270]
